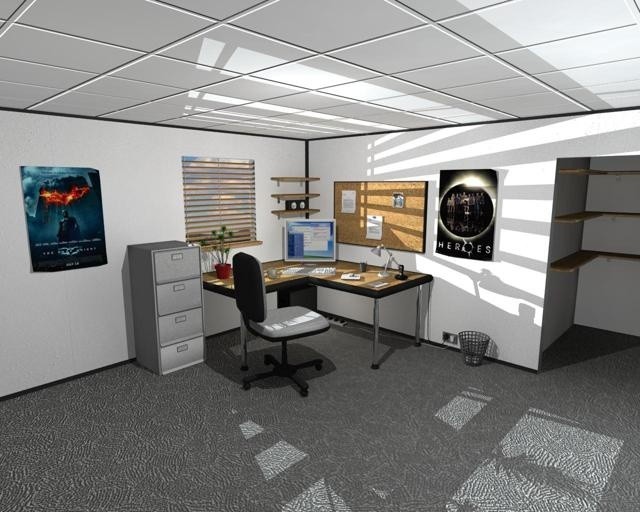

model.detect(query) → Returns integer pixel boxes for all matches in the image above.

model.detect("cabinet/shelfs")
[270,177,321,220]
[550,169,640,273]
[127,240,207,376]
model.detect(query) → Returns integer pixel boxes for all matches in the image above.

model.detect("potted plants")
[202,226,234,280]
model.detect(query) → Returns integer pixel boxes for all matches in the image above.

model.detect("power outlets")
[442,332,458,346]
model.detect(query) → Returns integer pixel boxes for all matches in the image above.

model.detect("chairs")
[232,253,331,398]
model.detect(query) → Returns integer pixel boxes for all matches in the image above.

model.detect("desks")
[202,259,433,371]
